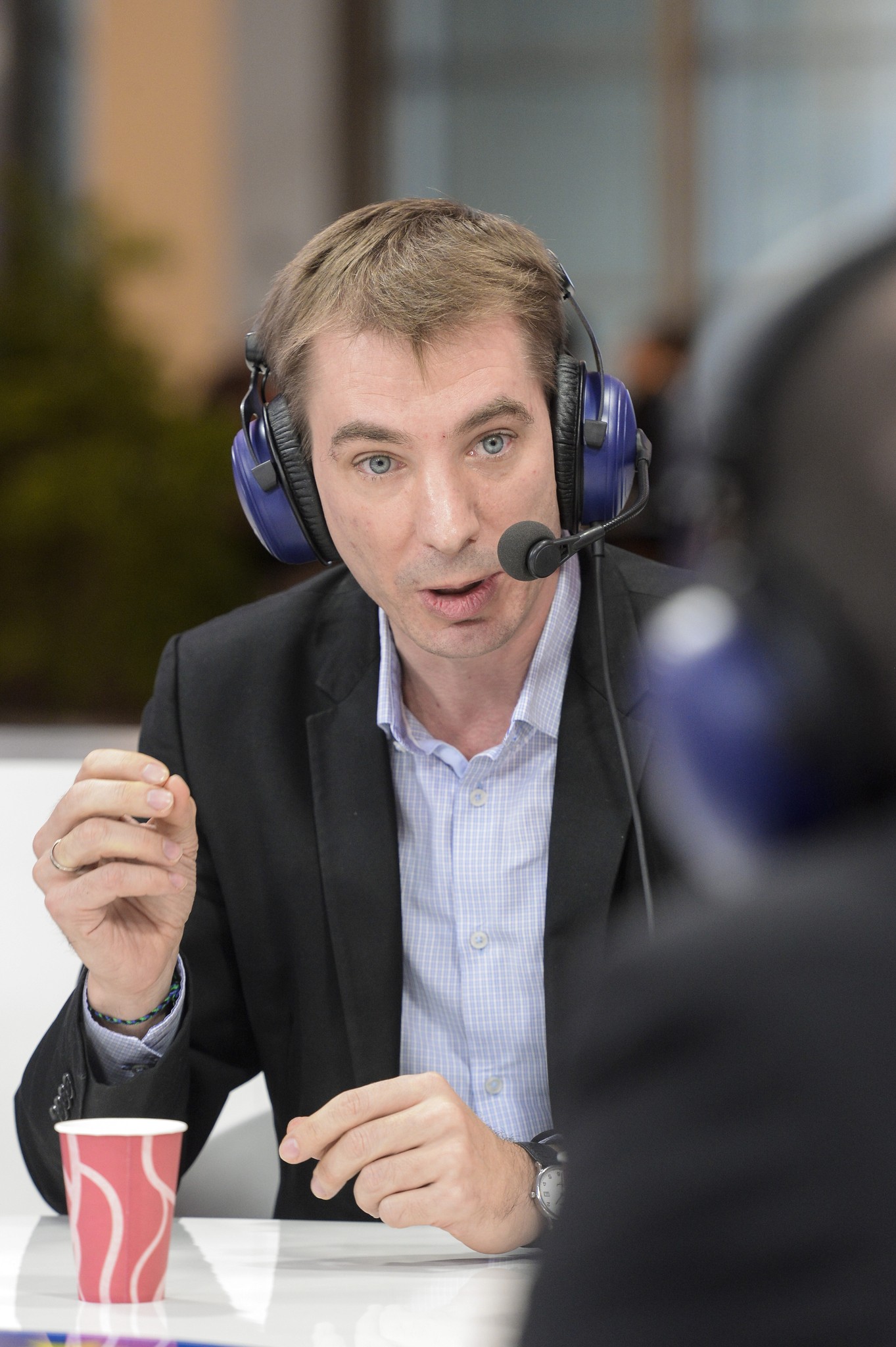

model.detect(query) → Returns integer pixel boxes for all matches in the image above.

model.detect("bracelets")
[86,973,181,1026]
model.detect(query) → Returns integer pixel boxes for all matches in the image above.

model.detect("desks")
[16,1209,539,1347]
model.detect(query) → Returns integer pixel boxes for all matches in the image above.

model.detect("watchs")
[512,1142,567,1249]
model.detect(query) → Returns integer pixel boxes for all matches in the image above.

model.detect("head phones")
[232,247,637,564]
[634,189,895,906]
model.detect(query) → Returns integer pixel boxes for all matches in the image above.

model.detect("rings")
[50,839,86,872]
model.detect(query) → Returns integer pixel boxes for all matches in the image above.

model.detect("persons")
[8,198,752,1258]
[508,186,896,1347]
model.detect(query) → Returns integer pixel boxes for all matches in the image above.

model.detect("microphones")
[497,428,653,581]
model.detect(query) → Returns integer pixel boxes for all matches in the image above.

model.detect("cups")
[52,1118,191,1301]
